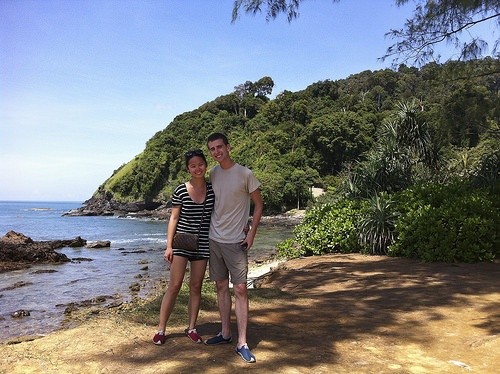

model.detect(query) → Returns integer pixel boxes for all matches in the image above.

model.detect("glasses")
[185,149,202,156]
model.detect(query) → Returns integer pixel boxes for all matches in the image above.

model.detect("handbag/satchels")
[172,233,199,251]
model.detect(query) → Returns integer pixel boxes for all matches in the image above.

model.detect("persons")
[153,149,249,345]
[207,133,264,363]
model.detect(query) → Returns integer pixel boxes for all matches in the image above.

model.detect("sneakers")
[185,327,202,343]
[203,332,233,346]
[152,330,166,345]
[235,343,256,363]
